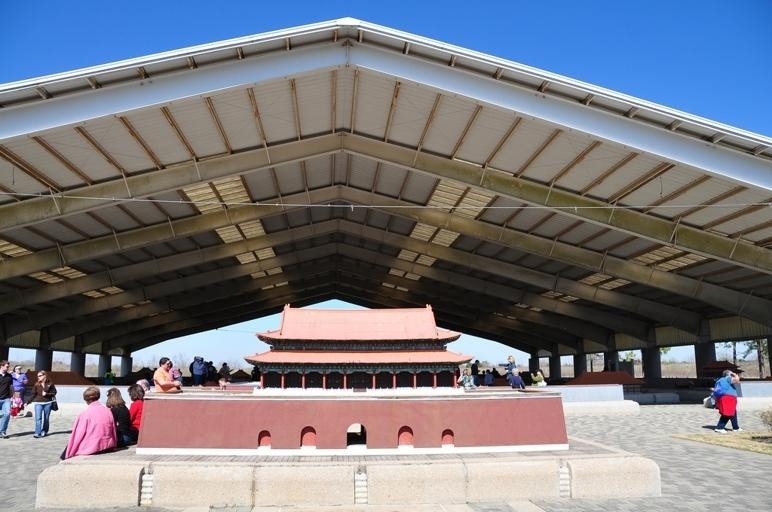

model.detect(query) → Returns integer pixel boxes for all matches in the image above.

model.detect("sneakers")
[33,430,47,438]
[732,427,746,433]
[714,428,729,434]
[0,432,9,438]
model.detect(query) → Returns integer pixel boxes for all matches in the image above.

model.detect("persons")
[531,369,544,386]
[457,360,500,392]
[25,370,57,438]
[499,356,526,389]
[711,370,745,433]
[0,360,29,439]
[190,356,230,385]
[60,357,182,463]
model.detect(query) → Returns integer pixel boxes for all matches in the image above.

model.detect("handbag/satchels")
[704,397,715,409]
[51,402,58,411]
